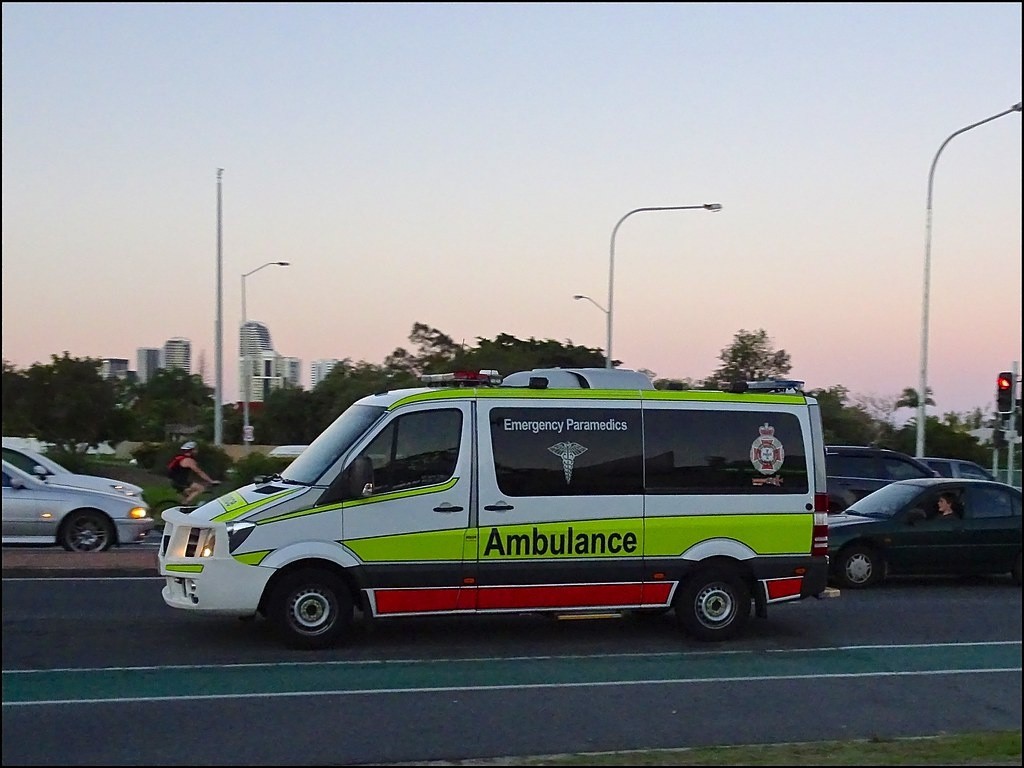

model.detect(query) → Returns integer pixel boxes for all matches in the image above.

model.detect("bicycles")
[150,483,221,530]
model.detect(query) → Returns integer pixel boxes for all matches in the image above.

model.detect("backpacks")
[167,456,197,479]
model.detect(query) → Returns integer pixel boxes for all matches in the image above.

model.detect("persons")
[936,492,957,518]
[165,442,221,506]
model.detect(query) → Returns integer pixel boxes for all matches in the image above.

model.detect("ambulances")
[160,366,831,649]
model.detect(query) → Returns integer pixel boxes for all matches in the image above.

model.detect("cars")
[912,458,996,481]
[2,459,153,552]
[3,445,145,502]
[825,443,941,513]
[831,476,1023,591]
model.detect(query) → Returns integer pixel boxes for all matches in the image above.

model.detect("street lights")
[240,262,291,452]
[573,203,723,367]
[914,101,1022,456]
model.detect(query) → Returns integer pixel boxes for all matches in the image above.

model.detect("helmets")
[180,442,198,453]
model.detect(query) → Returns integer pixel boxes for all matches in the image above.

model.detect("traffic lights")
[996,371,1016,415]
